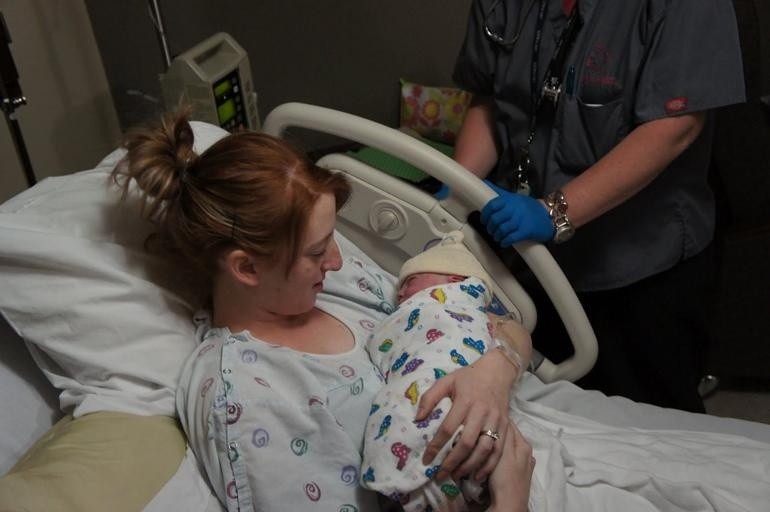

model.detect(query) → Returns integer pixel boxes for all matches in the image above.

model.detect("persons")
[433,2,746,414]
[114,89,540,511]
[394,225,496,308]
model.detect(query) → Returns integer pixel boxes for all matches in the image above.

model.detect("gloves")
[481,179,556,249]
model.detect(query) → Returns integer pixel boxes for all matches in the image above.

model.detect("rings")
[480,429,499,441]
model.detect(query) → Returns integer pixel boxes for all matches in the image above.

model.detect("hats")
[397,231,492,296]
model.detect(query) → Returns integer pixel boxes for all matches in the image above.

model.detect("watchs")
[543,190,576,245]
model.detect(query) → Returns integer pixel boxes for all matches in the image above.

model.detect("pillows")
[0,120,338,512]
[397,79,471,149]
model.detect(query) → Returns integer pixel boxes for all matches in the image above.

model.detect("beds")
[0,100,770,511]
